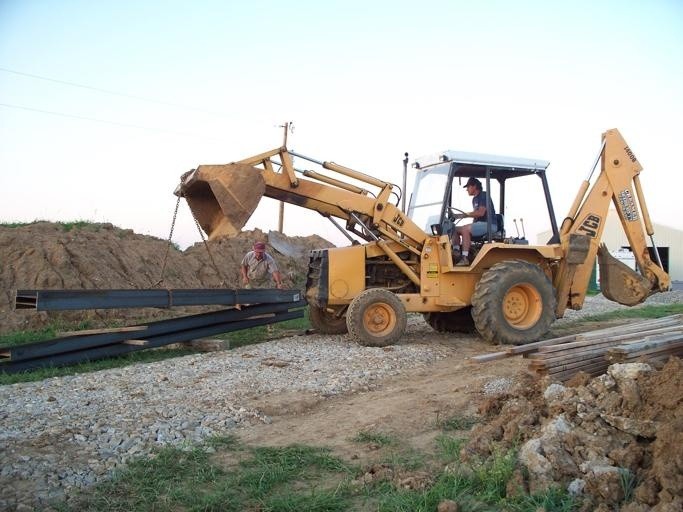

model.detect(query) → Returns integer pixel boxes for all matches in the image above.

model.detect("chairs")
[472,214,506,245]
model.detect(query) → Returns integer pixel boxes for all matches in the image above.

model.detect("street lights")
[402,152,408,213]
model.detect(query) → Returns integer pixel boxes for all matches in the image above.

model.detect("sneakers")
[452,249,469,265]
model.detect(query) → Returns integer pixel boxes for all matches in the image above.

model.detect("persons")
[240,242,283,307]
[448,176,498,267]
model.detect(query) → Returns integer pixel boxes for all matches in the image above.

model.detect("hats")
[463,176,482,187]
[254,242,265,253]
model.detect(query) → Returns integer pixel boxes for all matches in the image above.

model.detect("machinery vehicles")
[173,128,673,345]
